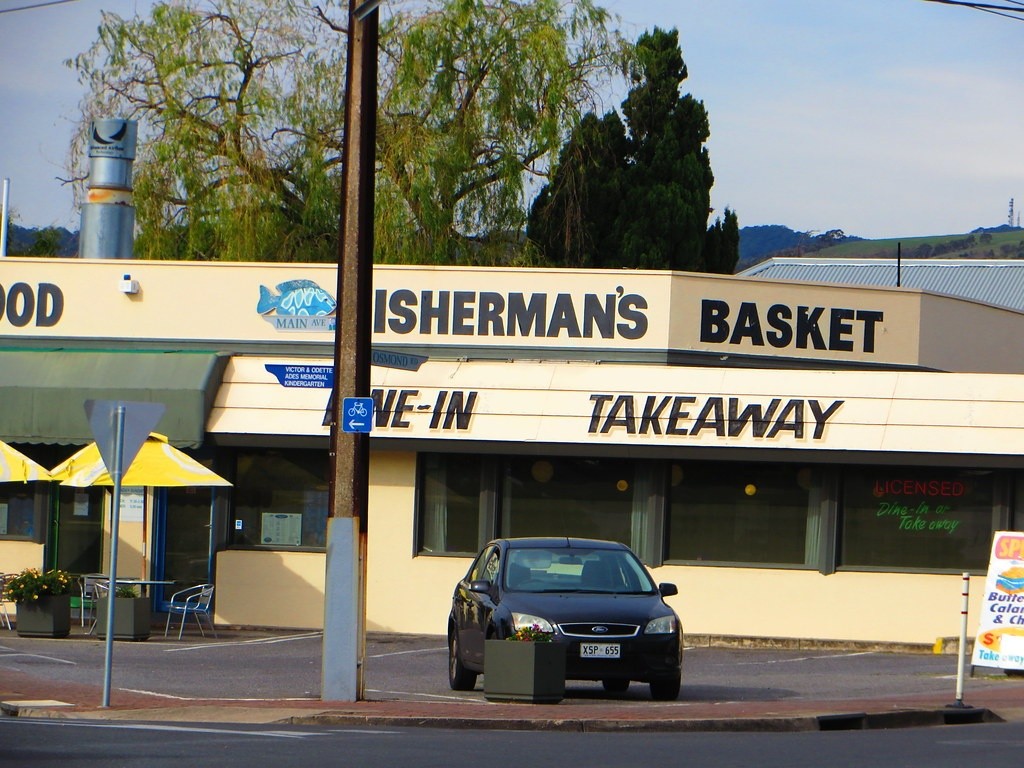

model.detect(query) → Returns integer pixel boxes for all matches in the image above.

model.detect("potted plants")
[96,583,152,642]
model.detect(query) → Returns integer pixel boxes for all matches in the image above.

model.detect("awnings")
[1,350,225,447]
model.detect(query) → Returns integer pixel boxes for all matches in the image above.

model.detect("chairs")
[580,560,614,588]
[509,563,531,588]
[164,583,218,640]
[77,573,108,628]
[67,575,83,628]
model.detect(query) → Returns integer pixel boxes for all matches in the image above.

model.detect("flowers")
[504,624,561,646]
[2,567,70,603]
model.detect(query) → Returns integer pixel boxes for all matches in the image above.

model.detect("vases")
[16,595,71,637]
[482,639,566,705]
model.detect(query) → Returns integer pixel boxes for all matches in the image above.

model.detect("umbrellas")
[0,432,233,581]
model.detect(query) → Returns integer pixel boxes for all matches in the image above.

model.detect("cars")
[446,534,685,702]
